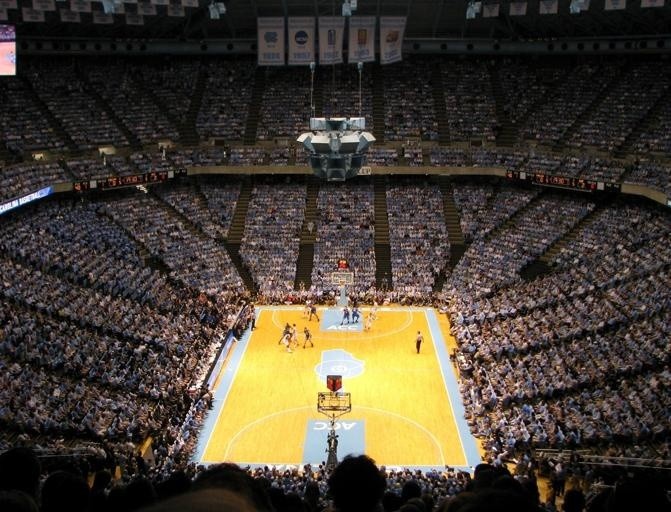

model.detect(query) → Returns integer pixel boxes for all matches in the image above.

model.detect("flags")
[286,15,317,65]
[463,0,664,20]
[257,16,284,66]
[346,15,376,63]
[378,15,406,65]
[0,0,198,27]
[318,15,346,64]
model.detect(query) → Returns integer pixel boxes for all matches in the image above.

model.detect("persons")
[371,299,380,320]
[278,322,294,345]
[0,52,670,306]
[303,326,314,349]
[340,306,351,325]
[365,313,378,333]
[436,306,671,512]
[0,307,256,512]
[291,323,300,348]
[255,455,435,512]
[352,306,363,323]
[284,333,293,352]
[307,303,321,322]
[415,330,425,354]
[303,298,313,318]
